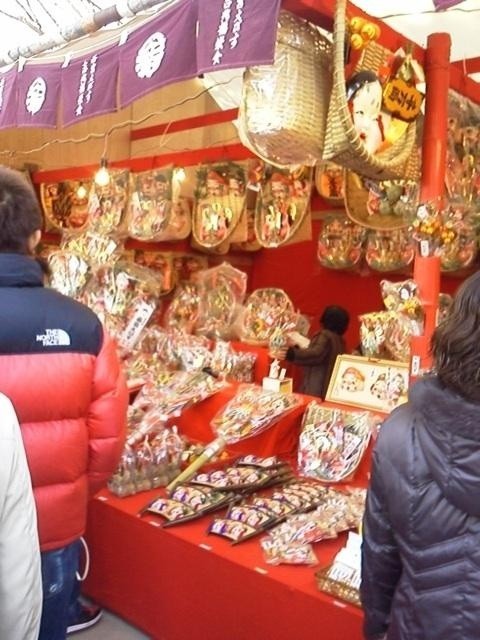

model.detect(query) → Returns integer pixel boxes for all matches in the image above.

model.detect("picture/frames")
[323,354,410,413]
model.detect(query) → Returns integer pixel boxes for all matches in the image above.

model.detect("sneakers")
[66,605,103,634]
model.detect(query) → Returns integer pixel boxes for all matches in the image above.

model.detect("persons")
[0,393,43,640]
[276,305,348,400]
[347,71,391,155]
[1,167,128,640]
[361,270,480,640]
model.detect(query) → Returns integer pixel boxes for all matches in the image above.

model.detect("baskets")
[314,565,363,609]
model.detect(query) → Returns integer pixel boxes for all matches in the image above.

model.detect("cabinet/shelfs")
[80,337,384,640]
